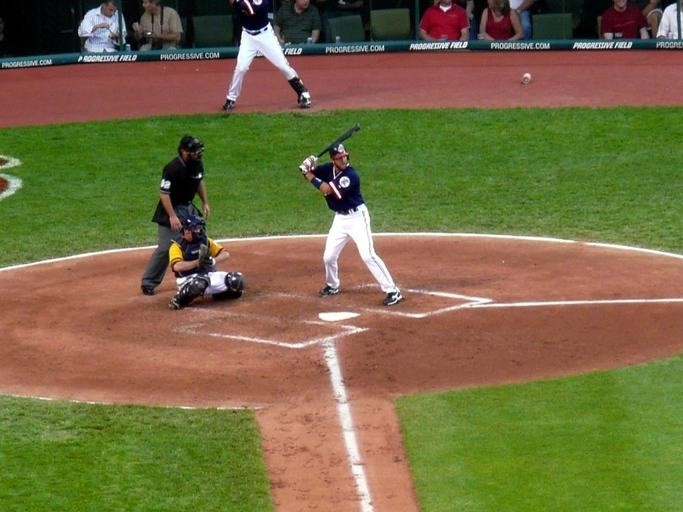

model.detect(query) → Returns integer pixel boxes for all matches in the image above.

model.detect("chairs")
[521,1,614,39]
[181,2,241,48]
[321,1,433,43]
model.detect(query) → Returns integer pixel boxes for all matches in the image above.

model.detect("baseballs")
[523,73,531,81]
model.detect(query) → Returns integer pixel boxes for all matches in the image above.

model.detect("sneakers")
[213,291,242,302]
[382,292,404,305]
[141,285,154,295]
[318,286,338,298]
[169,294,184,310]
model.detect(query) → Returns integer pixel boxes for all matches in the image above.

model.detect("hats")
[180,135,204,152]
[329,144,349,159]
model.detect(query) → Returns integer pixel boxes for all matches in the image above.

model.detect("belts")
[337,208,359,215]
[244,28,267,35]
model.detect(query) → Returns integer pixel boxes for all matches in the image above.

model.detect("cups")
[603,32,624,41]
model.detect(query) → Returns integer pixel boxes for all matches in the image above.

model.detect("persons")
[132,0,183,51]
[301,143,403,306]
[140,135,210,296]
[167,214,246,310]
[222,0,312,110]
[78,0,128,53]
[417,0,683,42]
[273,0,322,45]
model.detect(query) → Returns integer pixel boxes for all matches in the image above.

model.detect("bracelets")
[310,176,323,189]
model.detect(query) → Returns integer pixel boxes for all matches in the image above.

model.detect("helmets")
[180,215,206,239]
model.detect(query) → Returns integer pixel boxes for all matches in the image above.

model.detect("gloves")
[298,90,312,107]
[306,155,318,170]
[299,161,311,174]
[222,98,236,111]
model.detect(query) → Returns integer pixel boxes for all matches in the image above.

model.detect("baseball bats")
[299,123,360,171]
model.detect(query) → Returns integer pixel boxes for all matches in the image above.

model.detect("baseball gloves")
[199,243,215,268]
